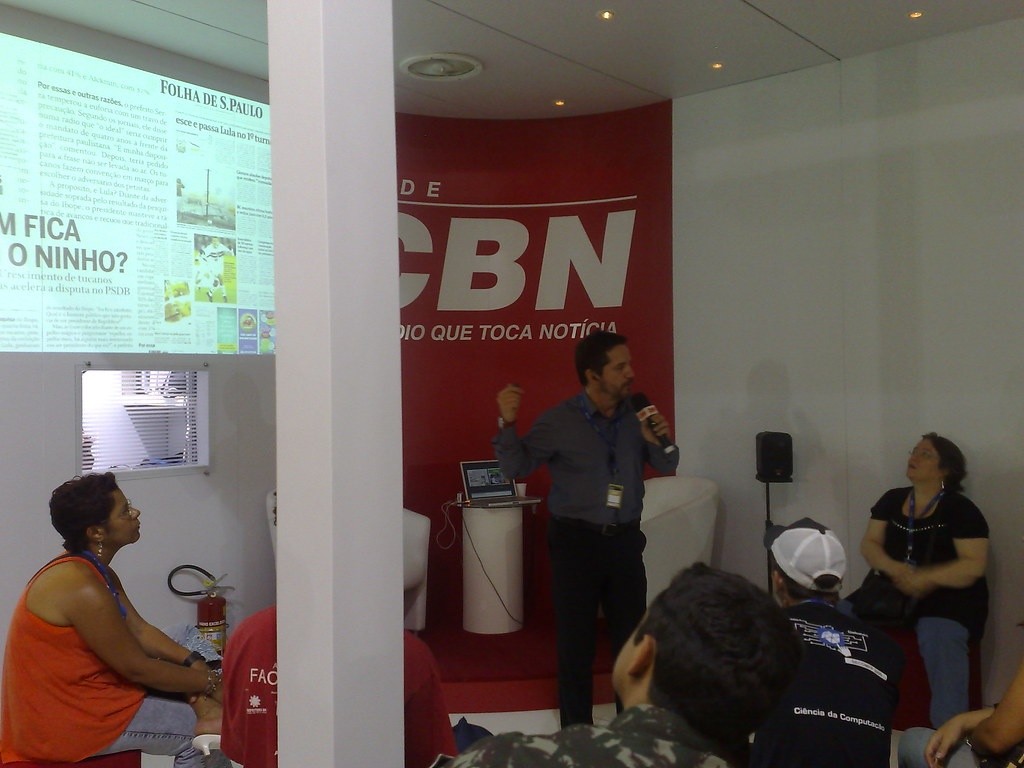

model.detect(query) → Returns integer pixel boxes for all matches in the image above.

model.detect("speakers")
[756,431,794,483]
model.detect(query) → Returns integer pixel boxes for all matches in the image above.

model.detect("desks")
[441,497,542,634]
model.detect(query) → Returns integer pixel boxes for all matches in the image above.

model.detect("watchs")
[183,650,206,667]
[497,416,518,429]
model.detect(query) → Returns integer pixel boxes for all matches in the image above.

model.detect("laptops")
[460,460,541,508]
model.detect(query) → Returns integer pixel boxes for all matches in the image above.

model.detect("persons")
[219,606,494,768]
[496,331,680,729]
[0,471,234,768]
[195,237,237,302]
[838,432,990,729]
[897,656,1024,768]
[746,518,896,768]
[439,560,805,768]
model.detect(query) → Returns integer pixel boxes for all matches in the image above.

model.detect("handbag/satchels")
[965,704,1024,768]
[852,576,919,629]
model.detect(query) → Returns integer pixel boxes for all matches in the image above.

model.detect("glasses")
[909,448,938,463]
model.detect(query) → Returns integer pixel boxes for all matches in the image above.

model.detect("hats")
[763,519,847,593]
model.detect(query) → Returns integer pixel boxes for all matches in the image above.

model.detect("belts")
[552,513,640,537]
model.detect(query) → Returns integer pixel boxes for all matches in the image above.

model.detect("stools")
[2,750,143,768]
[870,622,983,731]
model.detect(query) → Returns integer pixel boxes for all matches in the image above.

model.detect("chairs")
[266,487,430,634]
[641,475,720,604]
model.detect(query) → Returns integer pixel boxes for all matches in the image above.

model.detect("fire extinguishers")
[196,573,228,671]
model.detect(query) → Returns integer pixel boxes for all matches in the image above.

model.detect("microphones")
[631,391,675,454]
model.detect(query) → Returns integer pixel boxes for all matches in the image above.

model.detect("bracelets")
[967,739,987,762]
[202,668,223,701]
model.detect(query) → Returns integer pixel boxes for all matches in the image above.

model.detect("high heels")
[192,735,221,756]
